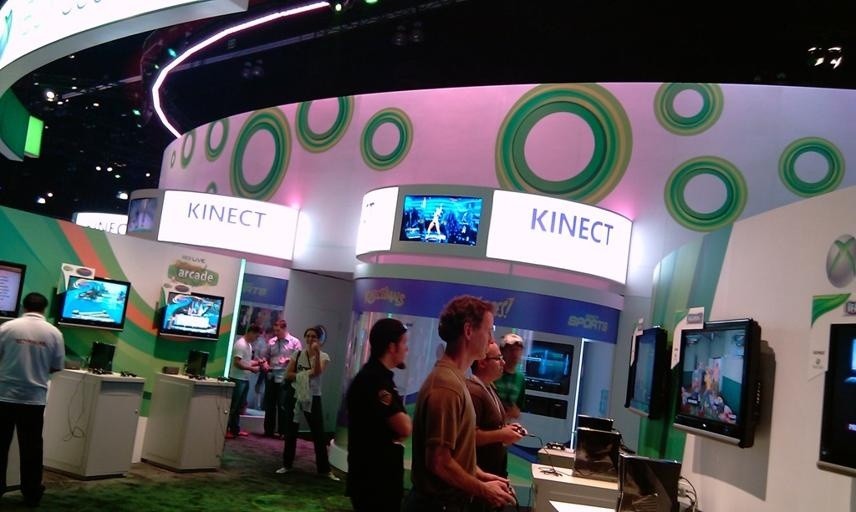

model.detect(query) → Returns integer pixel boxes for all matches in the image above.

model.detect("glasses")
[487,355,504,360]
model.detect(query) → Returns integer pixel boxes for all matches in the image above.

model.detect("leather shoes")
[327,472,340,481]
[275,468,289,473]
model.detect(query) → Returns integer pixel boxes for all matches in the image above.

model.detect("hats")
[500,334,524,349]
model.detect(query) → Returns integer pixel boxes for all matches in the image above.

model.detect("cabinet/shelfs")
[527,435,625,512]
[41,366,147,479]
[142,373,239,473]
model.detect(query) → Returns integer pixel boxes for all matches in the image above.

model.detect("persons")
[254,320,302,440]
[227,321,264,439]
[254,325,275,410]
[348,317,412,507]
[425,204,444,236]
[464,340,528,478]
[1,291,65,509]
[405,296,517,511]
[493,333,527,418]
[274,326,344,480]
[679,358,737,424]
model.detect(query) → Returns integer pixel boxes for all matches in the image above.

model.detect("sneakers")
[226,433,234,438]
[238,432,248,436]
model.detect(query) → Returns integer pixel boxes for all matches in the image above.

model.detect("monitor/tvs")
[126,198,157,232]
[55,275,131,331]
[672,318,763,449]
[816,323,856,477]
[624,328,672,419]
[524,340,574,395]
[0,260,27,319]
[399,194,482,246]
[160,292,224,341]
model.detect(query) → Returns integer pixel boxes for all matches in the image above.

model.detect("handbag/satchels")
[277,377,293,410]
